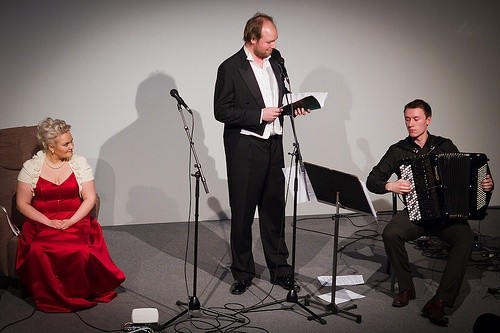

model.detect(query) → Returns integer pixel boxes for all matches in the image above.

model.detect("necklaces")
[44,158,66,169]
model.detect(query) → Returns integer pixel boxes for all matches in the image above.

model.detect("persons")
[366,100,494,325]
[15,118,126,313]
[214,12,311,295]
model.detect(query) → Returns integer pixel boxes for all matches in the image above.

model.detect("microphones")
[170,89,193,114]
[271,49,288,77]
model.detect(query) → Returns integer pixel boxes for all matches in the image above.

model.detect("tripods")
[155,171,246,331]
[241,150,328,325]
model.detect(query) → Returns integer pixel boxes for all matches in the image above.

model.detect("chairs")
[0,125,101,299]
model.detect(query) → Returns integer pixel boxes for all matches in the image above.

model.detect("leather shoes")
[392,284,415,306]
[270,276,300,292]
[230,277,251,294]
[422,295,449,326]
